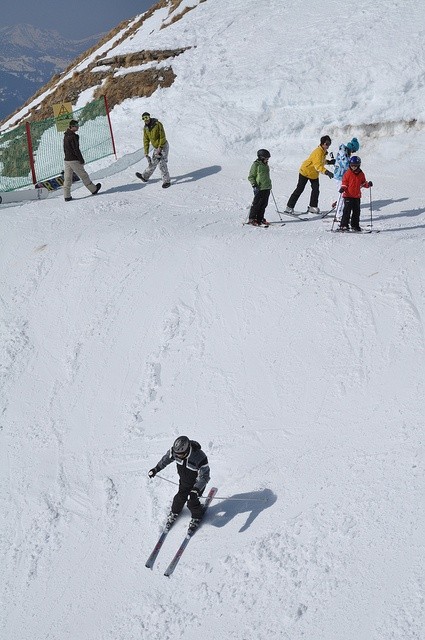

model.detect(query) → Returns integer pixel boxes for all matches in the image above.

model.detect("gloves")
[153,148,160,155]
[253,184,258,195]
[327,158,336,164]
[324,170,334,179]
[185,489,198,502]
[147,468,157,478]
[146,155,152,166]
[338,188,345,193]
[367,181,373,187]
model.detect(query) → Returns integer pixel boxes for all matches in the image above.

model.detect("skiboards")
[276,209,333,222]
[323,209,337,220]
[243,220,286,229]
[326,226,381,234]
[145,487,218,576]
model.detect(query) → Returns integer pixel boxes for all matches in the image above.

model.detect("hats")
[346,137,360,152]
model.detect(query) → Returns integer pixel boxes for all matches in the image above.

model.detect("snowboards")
[35,172,81,192]
[0,188,49,204]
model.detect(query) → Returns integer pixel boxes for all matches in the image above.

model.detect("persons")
[135,112,171,188]
[148,436,210,530]
[248,149,272,227]
[339,156,373,231]
[334,138,360,222]
[64,120,101,201]
[284,135,336,213]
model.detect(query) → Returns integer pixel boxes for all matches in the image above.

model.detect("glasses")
[324,142,330,146]
[68,123,79,128]
[141,116,150,121]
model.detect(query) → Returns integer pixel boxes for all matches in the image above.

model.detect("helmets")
[257,148,271,158]
[172,435,189,453]
[349,156,361,166]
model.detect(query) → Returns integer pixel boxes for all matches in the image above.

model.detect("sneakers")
[64,196,72,201]
[350,225,362,232]
[188,518,200,529]
[339,225,349,231]
[307,206,320,214]
[91,182,101,194]
[247,218,260,227]
[162,183,171,188]
[167,510,178,523]
[259,218,270,225]
[285,206,294,215]
[135,172,147,182]
